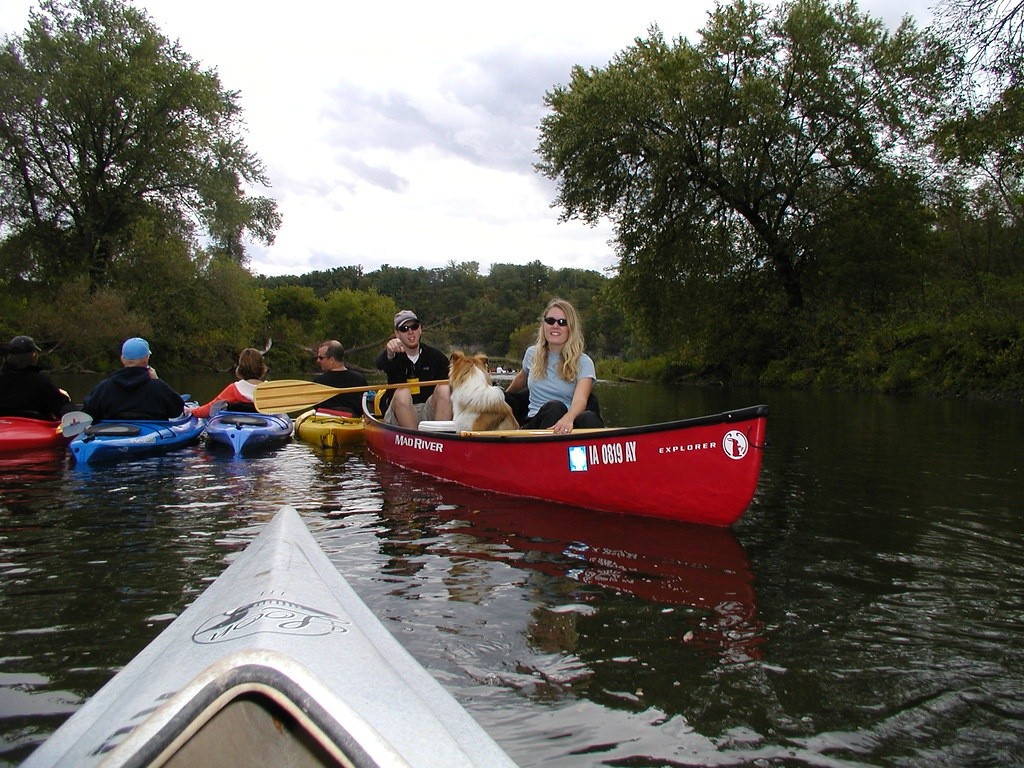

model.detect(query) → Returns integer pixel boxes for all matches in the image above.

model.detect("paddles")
[60,411,98,438]
[249,379,458,416]
[461,428,629,437]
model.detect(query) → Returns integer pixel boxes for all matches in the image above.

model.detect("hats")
[122,337,152,360]
[394,310,418,328]
[9,336,41,352]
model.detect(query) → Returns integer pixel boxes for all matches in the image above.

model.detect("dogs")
[449,351,519,431]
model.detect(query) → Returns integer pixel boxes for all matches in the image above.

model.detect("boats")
[69,401,207,468]
[294,407,367,453]
[362,392,769,528]
[0,416,61,454]
[205,400,292,456]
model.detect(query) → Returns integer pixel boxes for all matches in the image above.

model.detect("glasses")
[318,356,331,360]
[398,323,420,332]
[544,317,567,326]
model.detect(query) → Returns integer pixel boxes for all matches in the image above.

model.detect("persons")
[312,340,368,418]
[186,348,267,418]
[79,338,184,421]
[0,336,77,422]
[508,302,605,435]
[375,310,452,429]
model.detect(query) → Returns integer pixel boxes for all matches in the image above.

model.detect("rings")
[565,429,568,432]
